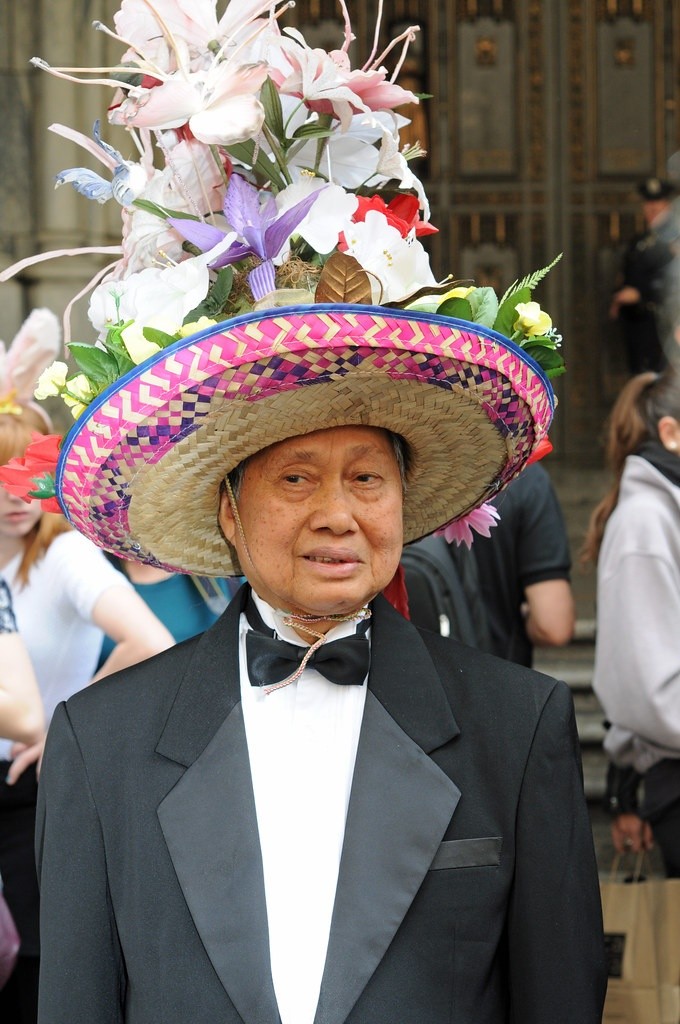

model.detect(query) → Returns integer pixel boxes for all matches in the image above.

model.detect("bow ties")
[247,628,372,690]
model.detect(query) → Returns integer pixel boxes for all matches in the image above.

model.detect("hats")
[0,0,560,578]
[635,178,679,200]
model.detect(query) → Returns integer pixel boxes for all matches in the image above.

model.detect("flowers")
[1,431,61,519]
[433,493,500,554]
[0,0,570,420]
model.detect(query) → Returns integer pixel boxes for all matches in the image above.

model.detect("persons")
[463,462,574,669]
[611,180,680,371]
[583,372,680,1024]
[39,425,608,1024]
[0,309,248,1023]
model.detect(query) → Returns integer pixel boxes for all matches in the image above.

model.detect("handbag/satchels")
[593,848,680,1024]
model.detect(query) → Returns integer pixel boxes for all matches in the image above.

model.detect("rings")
[624,837,632,845]
